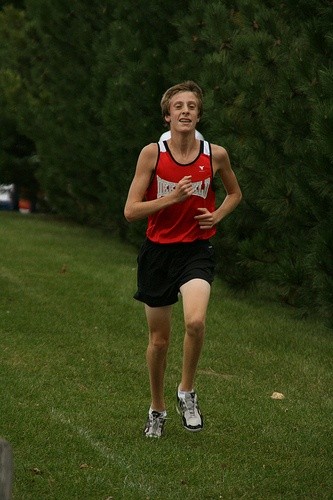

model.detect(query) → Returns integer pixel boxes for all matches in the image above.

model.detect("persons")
[124,81,243,438]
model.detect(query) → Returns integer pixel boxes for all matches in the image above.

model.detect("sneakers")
[175,386,202,431]
[141,408,167,440]
